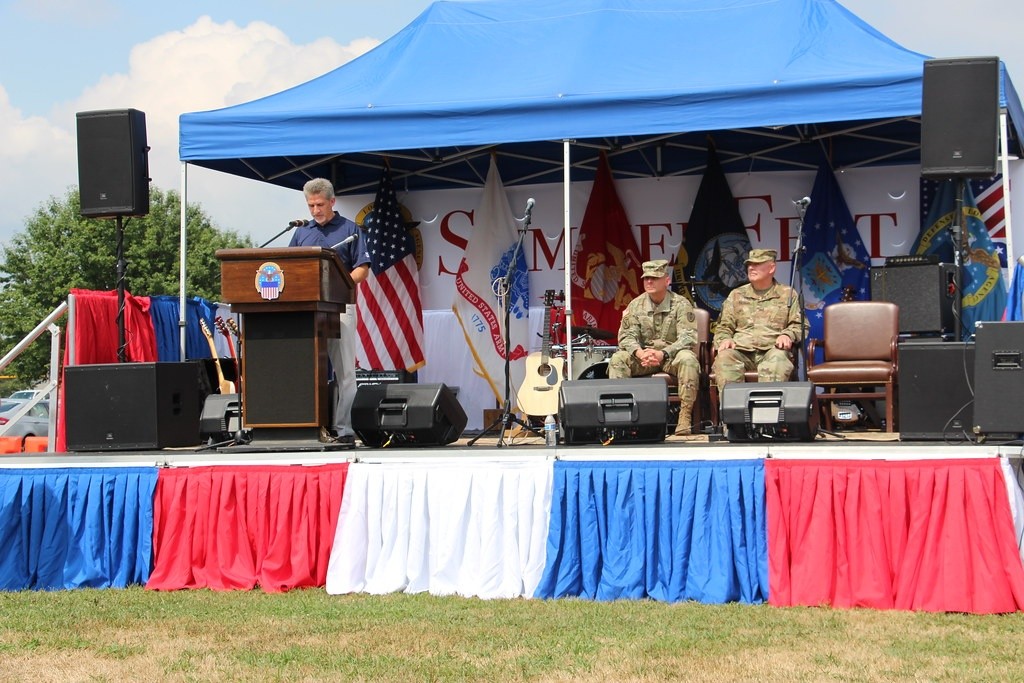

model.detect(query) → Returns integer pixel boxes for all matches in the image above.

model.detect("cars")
[0,398,49,438]
[10,391,50,400]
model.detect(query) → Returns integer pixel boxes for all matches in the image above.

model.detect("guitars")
[516,289,566,417]
[200,318,236,394]
[214,315,243,393]
[822,285,868,424]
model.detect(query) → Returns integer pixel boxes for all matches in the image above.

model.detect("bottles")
[545,413,556,447]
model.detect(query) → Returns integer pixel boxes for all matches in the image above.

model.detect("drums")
[577,357,611,379]
[571,351,608,380]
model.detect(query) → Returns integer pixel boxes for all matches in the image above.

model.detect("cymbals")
[560,326,615,339]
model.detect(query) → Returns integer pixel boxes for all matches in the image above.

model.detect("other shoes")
[721,421,729,438]
[335,435,356,449]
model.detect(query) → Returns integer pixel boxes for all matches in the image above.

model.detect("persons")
[606,260,702,436]
[711,249,811,437]
[289,178,372,444]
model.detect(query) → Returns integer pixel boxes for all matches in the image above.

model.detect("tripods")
[466,210,546,449]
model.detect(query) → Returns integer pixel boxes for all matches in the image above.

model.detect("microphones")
[289,220,309,227]
[331,234,358,249]
[526,198,535,214]
[796,197,811,206]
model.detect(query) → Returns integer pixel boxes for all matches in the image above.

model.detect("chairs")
[634,307,710,432]
[807,302,899,432]
[708,314,796,430]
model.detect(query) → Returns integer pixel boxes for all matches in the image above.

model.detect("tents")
[179,0,1024,364]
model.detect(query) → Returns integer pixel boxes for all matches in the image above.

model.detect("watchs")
[662,350,669,360]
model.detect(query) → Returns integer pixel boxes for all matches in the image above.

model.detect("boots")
[675,401,693,435]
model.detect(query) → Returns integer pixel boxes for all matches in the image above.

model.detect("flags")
[1007,263,1024,321]
[909,177,1007,343]
[570,149,645,346]
[452,159,530,414]
[789,129,871,364]
[671,133,753,322]
[356,162,427,373]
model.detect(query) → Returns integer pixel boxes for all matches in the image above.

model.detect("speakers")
[64,360,202,452]
[557,377,668,446]
[332,369,415,404]
[973,321,1024,433]
[721,382,822,442]
[351,383,468,449]
[920,55,999,178]
[896,343,974,440]
[868,263,957,335]
[76,108,152,219]
[200,393,253,449]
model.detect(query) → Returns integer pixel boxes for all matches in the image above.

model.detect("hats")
[640,259,669,278]
[743,249,778,263]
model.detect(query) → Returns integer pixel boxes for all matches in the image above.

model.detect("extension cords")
[708,433,728,441]
